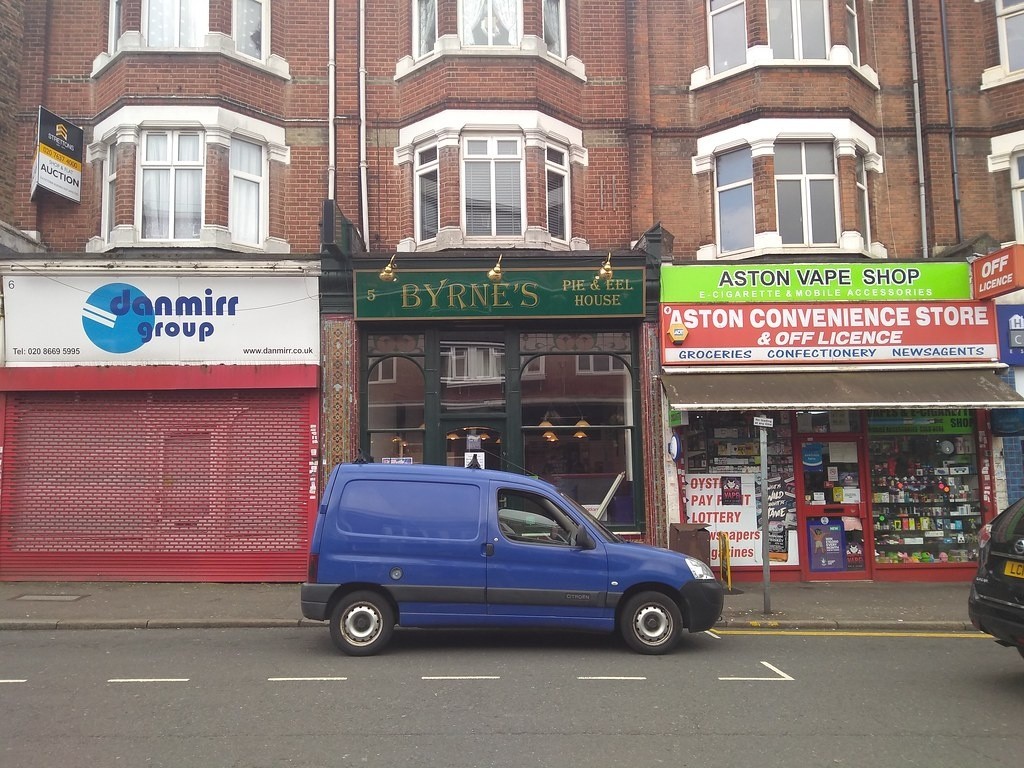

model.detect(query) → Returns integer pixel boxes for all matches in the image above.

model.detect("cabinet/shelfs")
[872,473,982,546]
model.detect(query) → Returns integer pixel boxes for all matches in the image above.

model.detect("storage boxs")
[934,468,949,475]
[949,467,969,474]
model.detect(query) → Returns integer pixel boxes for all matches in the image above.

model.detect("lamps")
[486,253,503,282]
[378,254,397,283]
[446,434,460,440]
[598,251,613,281]
[538,421,552,426]
[542,431,559,442]
[575,420,590,426]
[572,431,588,439]
[479,433,490,440]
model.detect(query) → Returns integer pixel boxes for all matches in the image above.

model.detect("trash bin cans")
[669,523,712,567]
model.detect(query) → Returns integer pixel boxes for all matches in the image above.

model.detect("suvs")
[968,494,1023,660]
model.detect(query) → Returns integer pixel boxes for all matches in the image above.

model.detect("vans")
[300,448,726,656]
